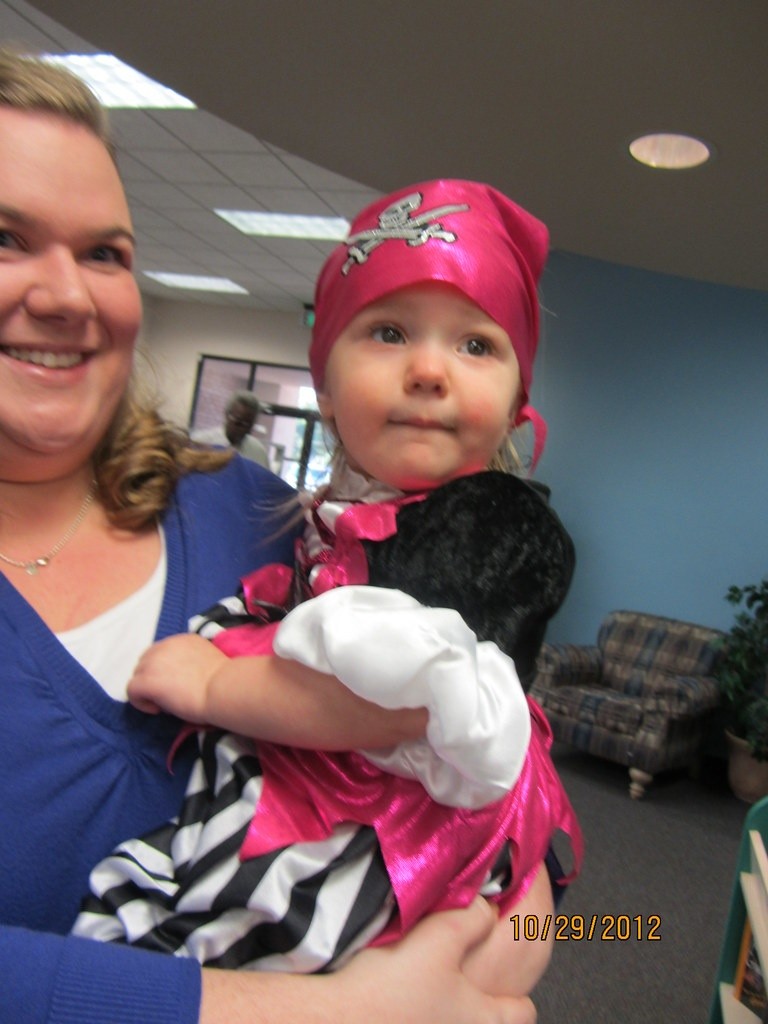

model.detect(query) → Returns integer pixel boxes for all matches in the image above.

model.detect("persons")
[0,50,566,1024]
[68,179,588,975]
[186,392,269,469]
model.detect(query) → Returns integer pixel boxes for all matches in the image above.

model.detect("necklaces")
[0,474,99,575]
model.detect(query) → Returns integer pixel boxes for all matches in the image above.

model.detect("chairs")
[527,609,730,801]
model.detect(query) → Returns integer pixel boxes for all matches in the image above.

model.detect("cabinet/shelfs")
[710,796,768,1024]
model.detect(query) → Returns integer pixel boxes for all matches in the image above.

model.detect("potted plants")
[703,579,768,804]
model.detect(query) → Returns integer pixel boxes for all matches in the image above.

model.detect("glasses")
[228,414,251,428]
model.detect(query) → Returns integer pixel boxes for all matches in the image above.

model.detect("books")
[732,918,768,1024]
